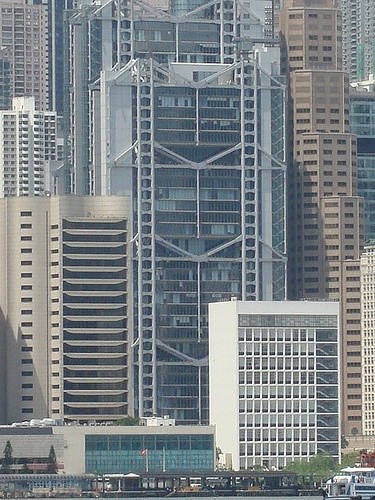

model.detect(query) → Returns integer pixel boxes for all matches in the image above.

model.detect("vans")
[327,467,375,485]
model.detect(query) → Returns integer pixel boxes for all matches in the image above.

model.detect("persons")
[318,486,326,500]
[107,482,111,491]
[166,486,184,496]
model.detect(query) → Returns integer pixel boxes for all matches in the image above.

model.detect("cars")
[25,473,326,492]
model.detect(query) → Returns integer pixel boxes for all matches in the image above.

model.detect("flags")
[140,449,147,456]
